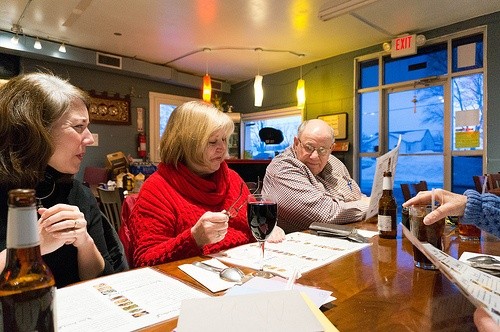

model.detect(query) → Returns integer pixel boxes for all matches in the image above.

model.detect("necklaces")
[35,181,55,207]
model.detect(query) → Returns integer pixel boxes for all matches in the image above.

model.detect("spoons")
[318,231,369,244]
[466,257,500,265]
[192,260,246,282]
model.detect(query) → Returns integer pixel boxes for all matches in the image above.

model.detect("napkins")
[459,252,500,270]
[357,229,380,237]
[178,257,244,293]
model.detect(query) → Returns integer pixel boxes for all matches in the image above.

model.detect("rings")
[73,219,79,228]
[216,232,221,238]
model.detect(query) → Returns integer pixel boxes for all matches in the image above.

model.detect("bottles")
[377,172,398,238]
[0,188,58,332]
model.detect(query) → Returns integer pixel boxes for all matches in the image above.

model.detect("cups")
[408,204,446,270]
[458,216,481,241]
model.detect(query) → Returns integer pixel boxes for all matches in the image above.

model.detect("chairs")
[400,171,500,203]
[97,187,122,230]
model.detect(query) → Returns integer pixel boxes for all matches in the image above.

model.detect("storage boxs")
[132,165,156,175]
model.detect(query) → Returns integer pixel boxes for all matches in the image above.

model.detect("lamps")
[202,48,212,103]
[11,31,19,45]
[253,48,264,107]
[58,41,66,53]
[318,0,379,21]
[34,36,42,49]
[296,54,305,109]
[383,33,426,58]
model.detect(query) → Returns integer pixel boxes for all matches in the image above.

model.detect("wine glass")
[248,193,278,271]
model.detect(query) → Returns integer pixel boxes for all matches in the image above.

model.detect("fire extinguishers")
[138,131,147,158]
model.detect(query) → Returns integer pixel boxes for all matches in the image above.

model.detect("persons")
[128,100,286,267]
[402,189,500,332]
[0,73,129,287]
[261,119,371,233]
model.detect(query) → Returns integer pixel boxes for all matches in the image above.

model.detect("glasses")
[297,138,331,156]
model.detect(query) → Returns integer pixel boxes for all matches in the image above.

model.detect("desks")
[66,216,500,332]
